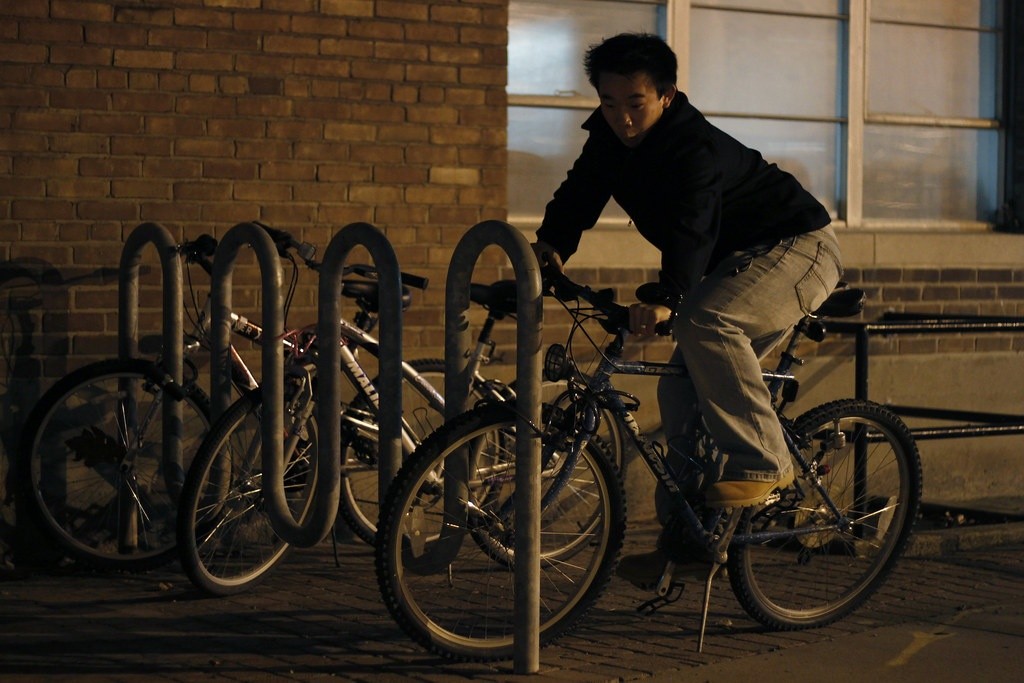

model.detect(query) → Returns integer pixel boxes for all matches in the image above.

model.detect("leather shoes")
[711,463,795,507]
[616,549,720,582]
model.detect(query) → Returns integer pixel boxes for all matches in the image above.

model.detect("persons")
[489,32,845,591]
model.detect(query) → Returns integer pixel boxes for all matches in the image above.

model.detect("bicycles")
[13,235,505,578]
[373,255,927,662]
[183,220,629,596]
